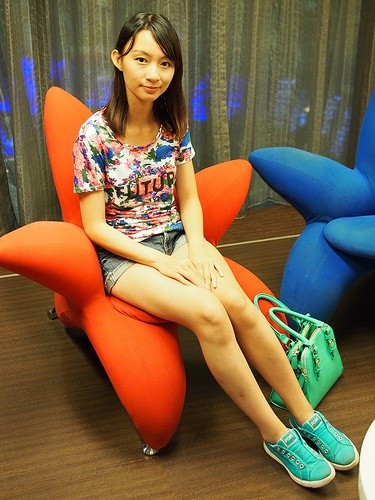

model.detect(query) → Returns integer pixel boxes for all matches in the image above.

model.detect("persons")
[72,12,360,488]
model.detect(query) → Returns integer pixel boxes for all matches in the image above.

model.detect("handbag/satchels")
[254,293,344,411]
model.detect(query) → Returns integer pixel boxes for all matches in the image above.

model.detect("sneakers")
[263,427,336,488]
[289,410,359,471]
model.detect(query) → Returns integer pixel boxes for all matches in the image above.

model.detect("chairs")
[248,88,374,341]
[0,85,291,455]
